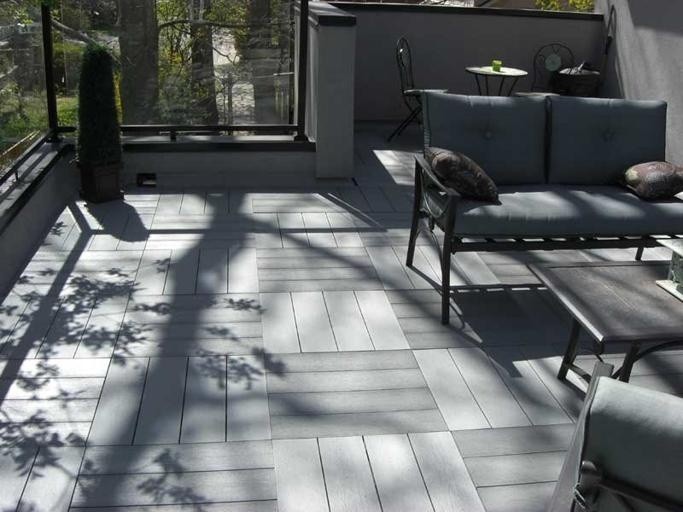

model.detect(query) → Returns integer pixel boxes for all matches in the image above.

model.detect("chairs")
[514,43,575,98]
[386,38,447,145]
[545,361,682,512]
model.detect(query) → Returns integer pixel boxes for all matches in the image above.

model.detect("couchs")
[404,89,683,325]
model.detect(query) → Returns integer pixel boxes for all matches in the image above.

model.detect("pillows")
[616,160,683,203]
[423,146,499,205]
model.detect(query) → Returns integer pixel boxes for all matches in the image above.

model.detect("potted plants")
[71,45,127,203]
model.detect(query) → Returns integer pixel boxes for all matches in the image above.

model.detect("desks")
[465,66,528,98]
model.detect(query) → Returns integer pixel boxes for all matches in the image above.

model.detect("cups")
[491,60,502,72]
